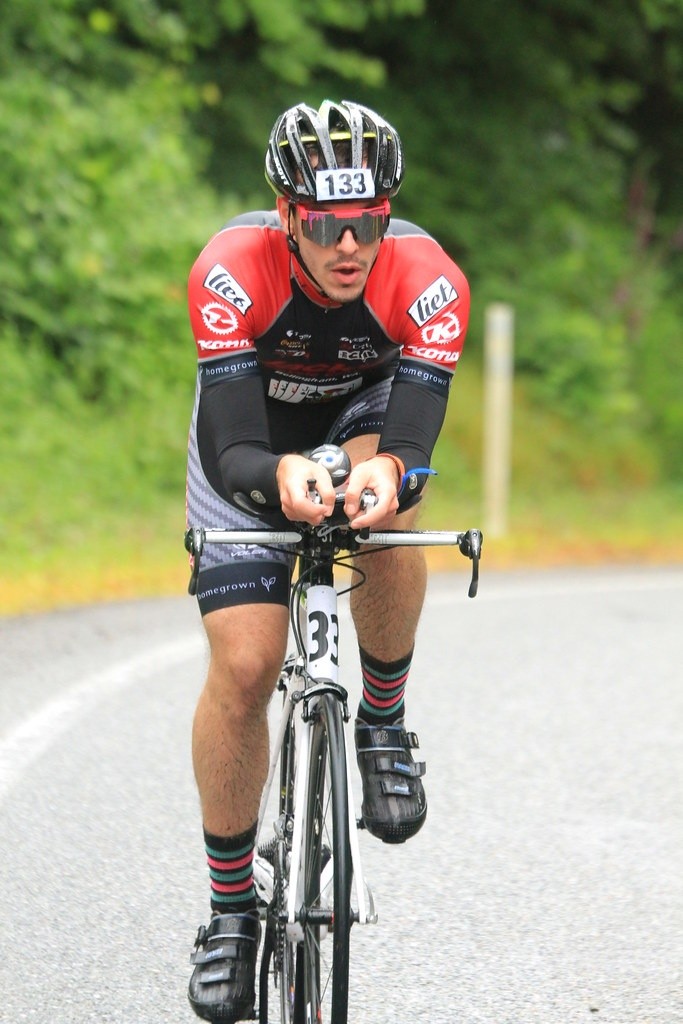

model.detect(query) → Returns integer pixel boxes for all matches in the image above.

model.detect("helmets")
[264,98,404,202]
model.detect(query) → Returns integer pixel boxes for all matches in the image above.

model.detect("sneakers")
[354,717,428,840]
[187,910,262,1024]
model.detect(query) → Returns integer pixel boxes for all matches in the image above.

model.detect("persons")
[185,100,470,1024]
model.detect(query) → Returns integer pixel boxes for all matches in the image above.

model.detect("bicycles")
[184,445,482,1023]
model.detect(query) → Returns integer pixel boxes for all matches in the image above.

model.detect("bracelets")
[366,455,401,492]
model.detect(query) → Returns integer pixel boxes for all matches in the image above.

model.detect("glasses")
[293,200,390,248]
